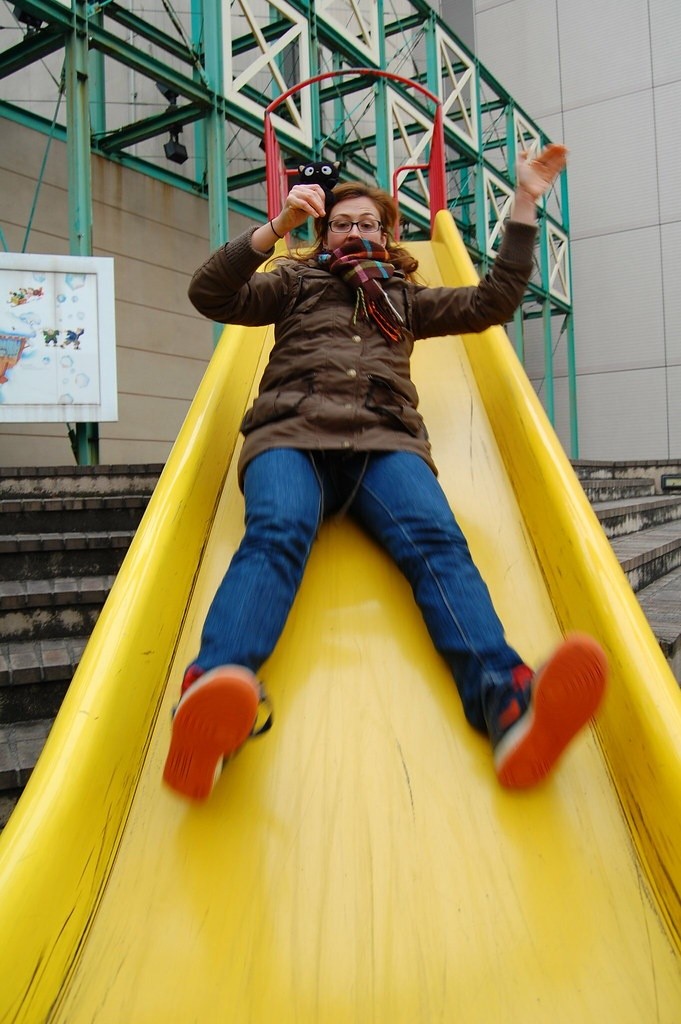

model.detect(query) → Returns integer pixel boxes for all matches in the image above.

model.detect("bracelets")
[270,219,284,239]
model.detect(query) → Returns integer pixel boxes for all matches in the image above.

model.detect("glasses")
[324,219,385,233]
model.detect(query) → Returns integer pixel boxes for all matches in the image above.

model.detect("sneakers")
[158,664,261,806]
[484,634,610,798]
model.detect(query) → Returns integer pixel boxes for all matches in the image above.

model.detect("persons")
[161,143,608,805]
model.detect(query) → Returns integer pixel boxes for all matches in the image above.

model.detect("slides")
[0,209,681,1023]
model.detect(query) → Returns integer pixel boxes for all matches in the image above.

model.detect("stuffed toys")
[298,160,343,205]
